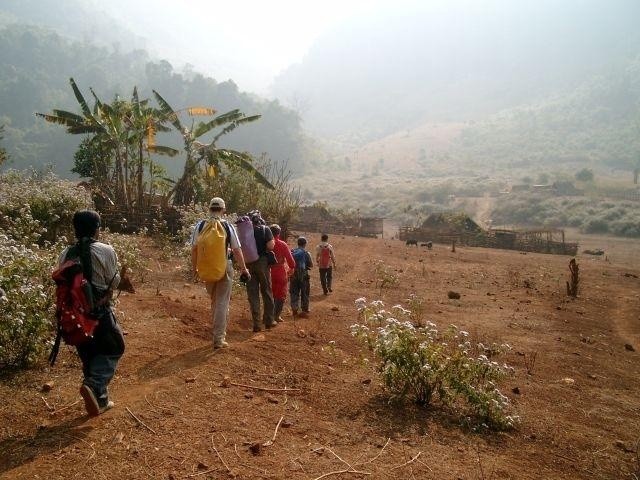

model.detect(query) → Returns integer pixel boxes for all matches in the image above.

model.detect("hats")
[209,197,225,209]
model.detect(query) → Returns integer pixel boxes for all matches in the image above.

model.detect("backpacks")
[235,216,260,264]
[293,248,305,271]
[52,240,110,346]
[198,219,228,280]
[319,244,330,268]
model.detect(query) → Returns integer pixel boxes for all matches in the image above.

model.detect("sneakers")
[213,341,227,348]
[80,383,100,416]
[98,401,114,413]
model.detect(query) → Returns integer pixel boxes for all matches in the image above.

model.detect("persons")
[270,222,296,322]
[290,235,313,315]
[237,209,277,330]
[191,197,252,349]
[49,209,133,416]
[314,234,337,294]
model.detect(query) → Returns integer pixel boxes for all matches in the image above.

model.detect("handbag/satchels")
[267,251,279,266]
